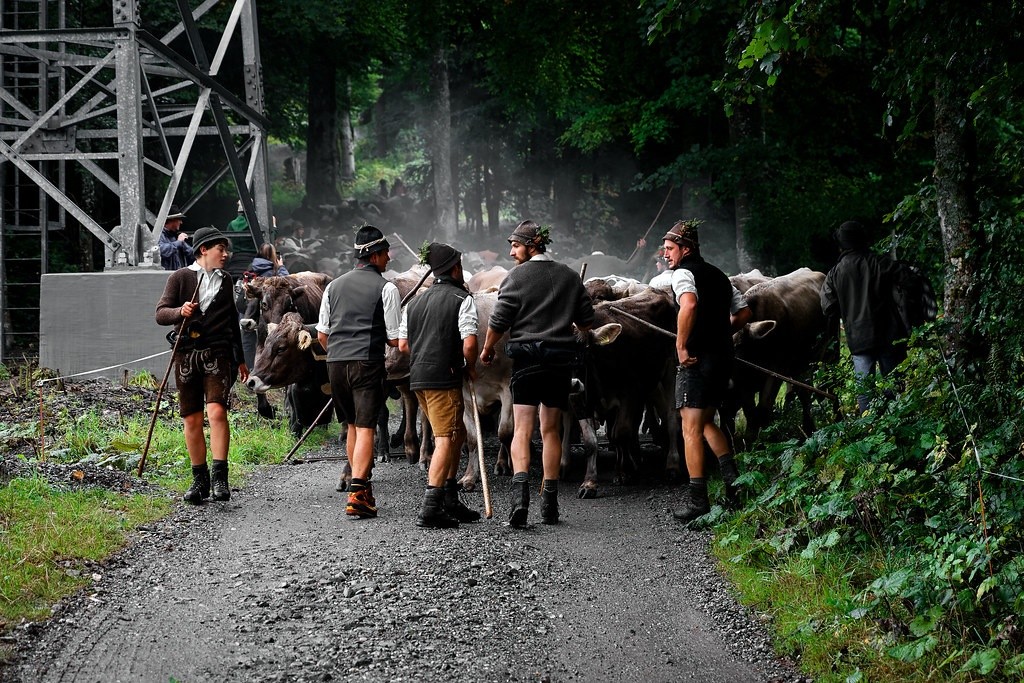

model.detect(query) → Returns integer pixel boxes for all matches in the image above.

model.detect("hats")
[838,222,863,246]
[662,221,700,247]
[193,228,228,256]
[292,221,303,233]
[652,245,668,263]
[427,243,462,276]
[354,226,390,258]
[167,205,186,219]
[238,199,253,212]
[507,220,547,252]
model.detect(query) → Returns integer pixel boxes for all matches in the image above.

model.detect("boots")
[211,460,231,499]
[541,479,560,524]
[714,455,748,505]
[674,478,710,520]
[417,486,459,528]
[184,464,211,500]
[509,472,530,527]
[444,484,482,523]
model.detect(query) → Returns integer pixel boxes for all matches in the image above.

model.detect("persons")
[284,221,304,249]
[377,177,402,198]
[241,242,289,371]
[398,243,480,528]
[386,235,403,273]
[571,238,645,284]
[156,228,248,500]
[158,205,194,270]
[314,226,402,517]
[227,198,277,276]
[649,246,674,288]
[480,220,594,523]
[820,222,921,418]
[662,221,749,522]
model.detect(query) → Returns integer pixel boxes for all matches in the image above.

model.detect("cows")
[244,270,334,364]
[391,260,835,494]
[245,312,331,440]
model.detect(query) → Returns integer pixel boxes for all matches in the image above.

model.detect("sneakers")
[346,484,379,516]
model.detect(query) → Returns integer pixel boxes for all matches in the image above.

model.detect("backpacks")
[233,263,273,314]
